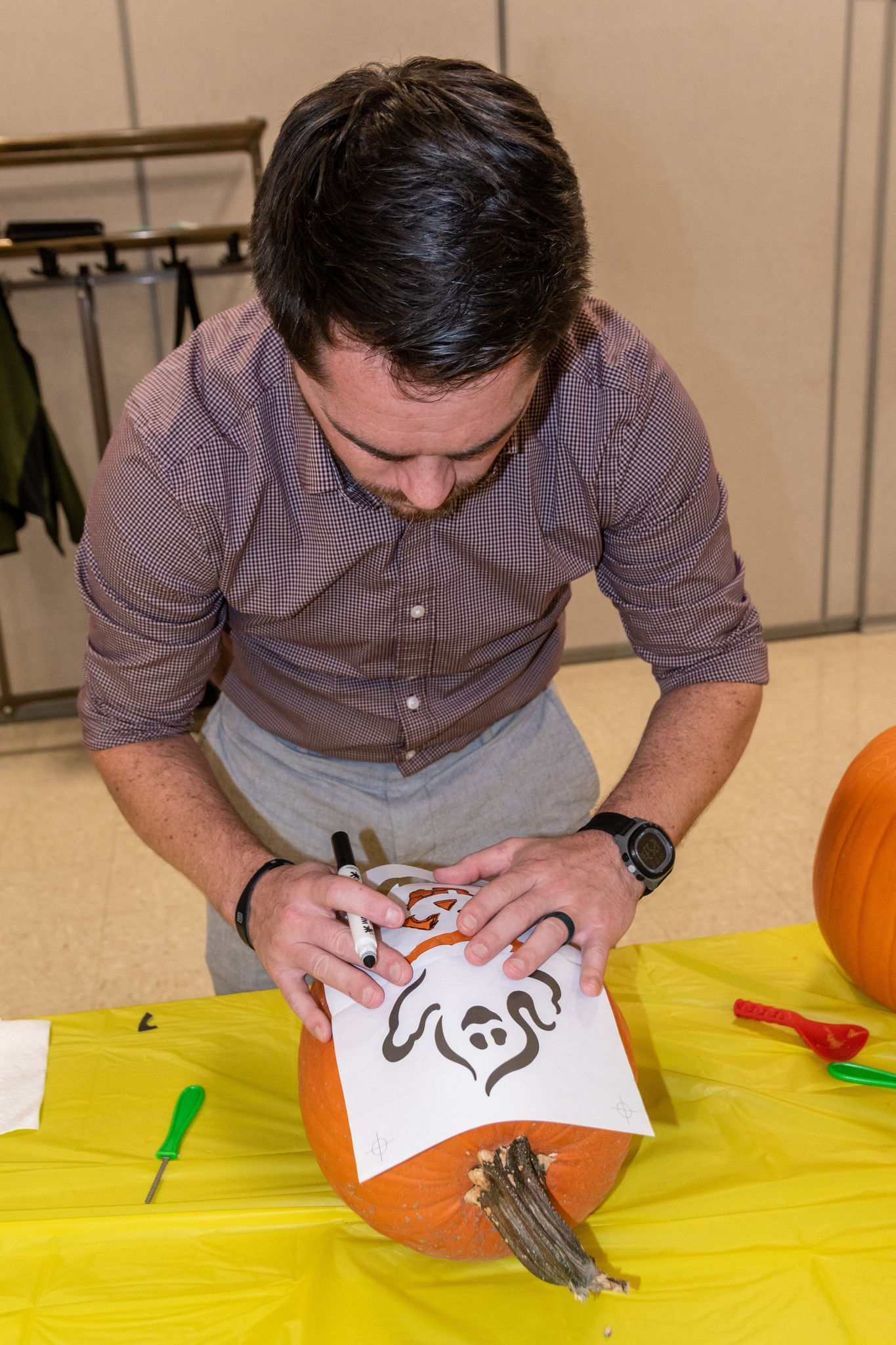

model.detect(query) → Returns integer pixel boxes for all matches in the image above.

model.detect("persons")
[67,51,779,1045]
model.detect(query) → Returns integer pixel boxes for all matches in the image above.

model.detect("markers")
[329,831,379,968]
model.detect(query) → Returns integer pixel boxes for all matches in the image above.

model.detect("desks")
[0,919,896,1345]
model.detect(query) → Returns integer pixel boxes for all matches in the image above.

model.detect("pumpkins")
[297,984,639,1300]
[812,725,896,1013]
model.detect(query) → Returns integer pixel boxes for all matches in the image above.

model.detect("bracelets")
[235,857,294,948]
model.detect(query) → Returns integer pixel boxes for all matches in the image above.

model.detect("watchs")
[574,809,676,900]
